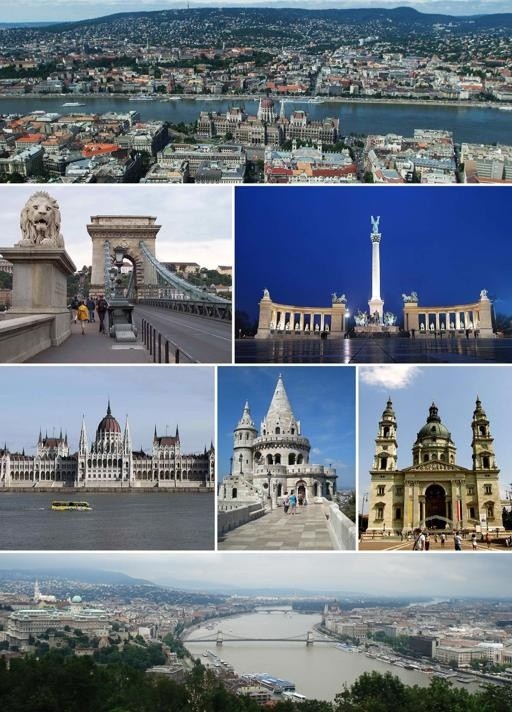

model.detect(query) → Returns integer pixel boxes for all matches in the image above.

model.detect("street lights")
[485,503,490,534]
[361,492,369,527]
[113,246,126,301]
[200,267,209,291]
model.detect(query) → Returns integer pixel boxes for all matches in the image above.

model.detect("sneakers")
[74,319,108,335]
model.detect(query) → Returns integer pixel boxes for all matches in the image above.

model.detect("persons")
[400,527,491,551]
[282,491,304,515]
[71,294,108,336]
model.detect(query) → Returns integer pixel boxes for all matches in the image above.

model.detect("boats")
[169,97,184,100]
[203,649,233,671]
[159,100,169,103]
[128,95,158,102]
[335,642,499,690]
[281,691,306,702]
[254,97,260,102]
[279,96,325,105]
[48,500,94,512]
[63,102,87,107]
[498,106,512,112]
